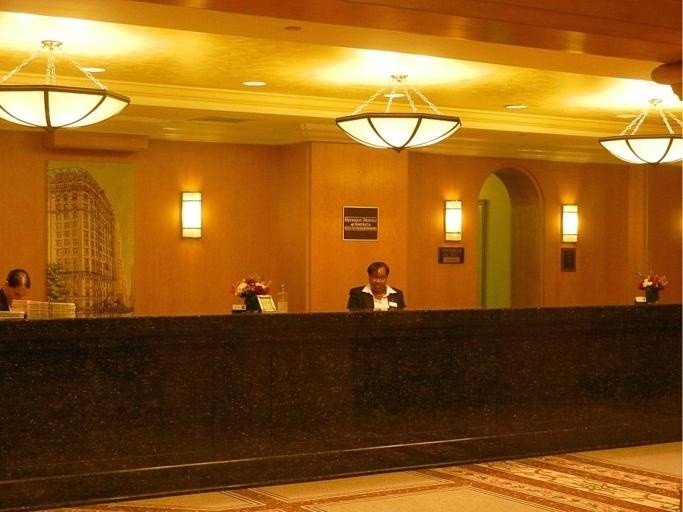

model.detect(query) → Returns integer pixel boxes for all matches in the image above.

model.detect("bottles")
[274,283,289,314]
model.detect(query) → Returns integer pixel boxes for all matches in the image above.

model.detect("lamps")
[562,205,578,242]
[335,74,462,154]
[598,98,683,166]
[0,39,130,134]
[181,192,201,238]
[445,200,462,242]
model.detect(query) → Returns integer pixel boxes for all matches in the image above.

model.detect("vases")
[244,295,262,312]
[645,288,659,304]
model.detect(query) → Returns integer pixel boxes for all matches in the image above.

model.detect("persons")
[0,269,30,311]
[347,262,406,311]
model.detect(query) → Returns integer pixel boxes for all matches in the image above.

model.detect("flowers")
[231,272,272,296]
[635,270,670,290]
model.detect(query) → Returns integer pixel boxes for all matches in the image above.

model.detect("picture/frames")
[342,206,379,241]
